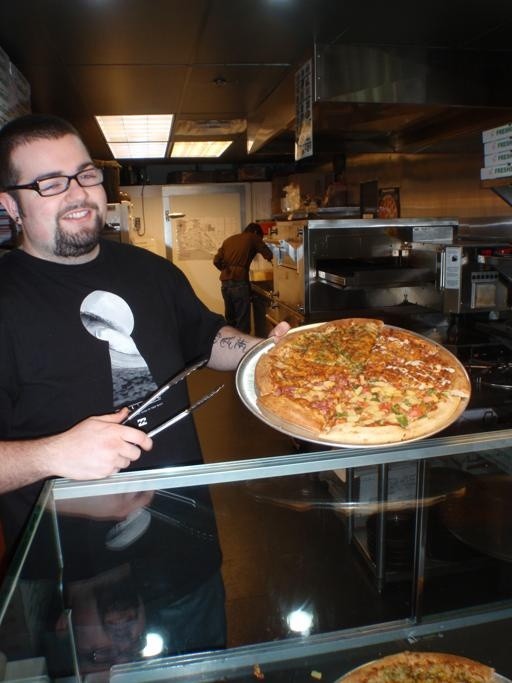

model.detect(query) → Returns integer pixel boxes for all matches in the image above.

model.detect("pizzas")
[337,651,495,683]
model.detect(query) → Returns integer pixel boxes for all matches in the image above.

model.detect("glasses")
[3,167,106,198]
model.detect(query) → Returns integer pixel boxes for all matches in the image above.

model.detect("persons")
[211,222,275,335]
[0,488,156,682]
[0,110,293,682]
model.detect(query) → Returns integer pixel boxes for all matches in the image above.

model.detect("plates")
[333,653,512,683]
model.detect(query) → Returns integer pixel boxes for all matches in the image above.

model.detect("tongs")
[120,359,225,445]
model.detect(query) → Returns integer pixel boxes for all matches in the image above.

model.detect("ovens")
[272,216,460,323]
